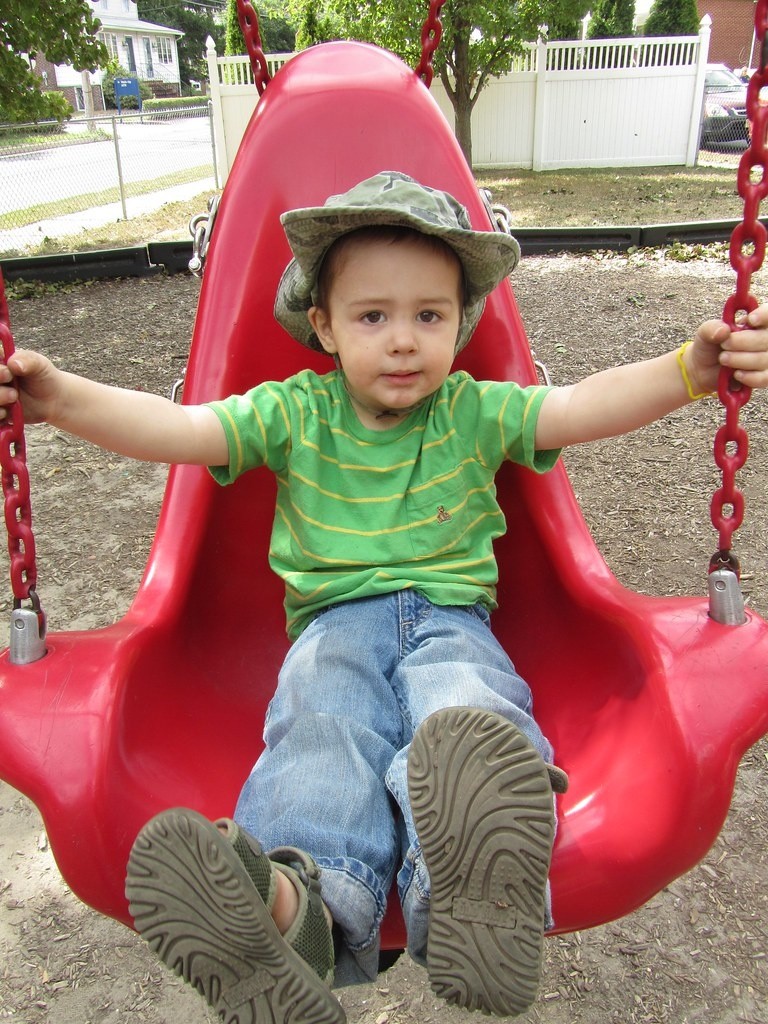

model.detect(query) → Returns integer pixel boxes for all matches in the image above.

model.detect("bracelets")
[677,337,715,399]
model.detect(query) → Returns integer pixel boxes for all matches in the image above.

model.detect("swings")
[1,2,768,957]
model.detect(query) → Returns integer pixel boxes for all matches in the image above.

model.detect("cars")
[697,62,751,150]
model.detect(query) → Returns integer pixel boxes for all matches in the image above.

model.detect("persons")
[0,173,768,1024]
[739,66,751,84]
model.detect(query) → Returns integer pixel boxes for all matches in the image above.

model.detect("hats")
[270,167,521,365]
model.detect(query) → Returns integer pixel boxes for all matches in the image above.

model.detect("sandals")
[404,704,570,1020]
[122,805,351,1024]
